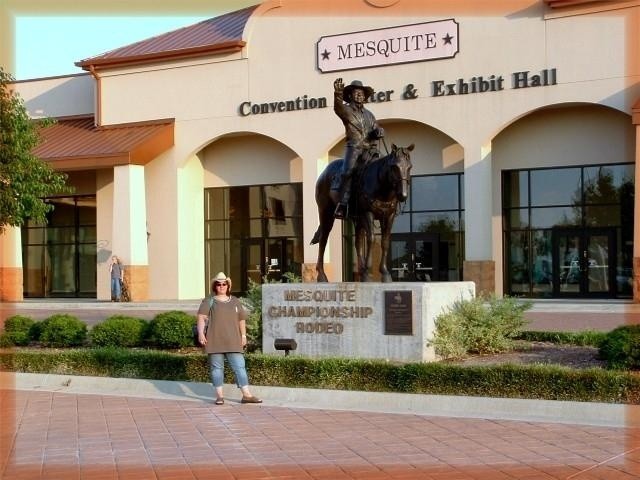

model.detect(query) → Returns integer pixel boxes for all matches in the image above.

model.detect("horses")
[308,142,416,284]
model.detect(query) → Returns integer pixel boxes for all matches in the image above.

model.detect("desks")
[561,263,608,290]
[389,267,432,282]
[246,268,280,283]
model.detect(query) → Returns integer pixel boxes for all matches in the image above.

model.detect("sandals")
[242,395,263,403]
[215,397,224,405]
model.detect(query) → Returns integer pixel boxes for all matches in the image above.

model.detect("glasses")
[216,283,227,286]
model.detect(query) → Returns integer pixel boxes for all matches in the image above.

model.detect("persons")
[197,272,261,404]
[334,78,384,218]
[109,255,123,302]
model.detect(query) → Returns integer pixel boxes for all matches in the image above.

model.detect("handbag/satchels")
[193,318,209,348]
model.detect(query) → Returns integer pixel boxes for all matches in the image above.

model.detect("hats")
[210,271,231,292]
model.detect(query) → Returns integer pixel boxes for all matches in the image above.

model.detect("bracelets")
[242,334,247,338]
